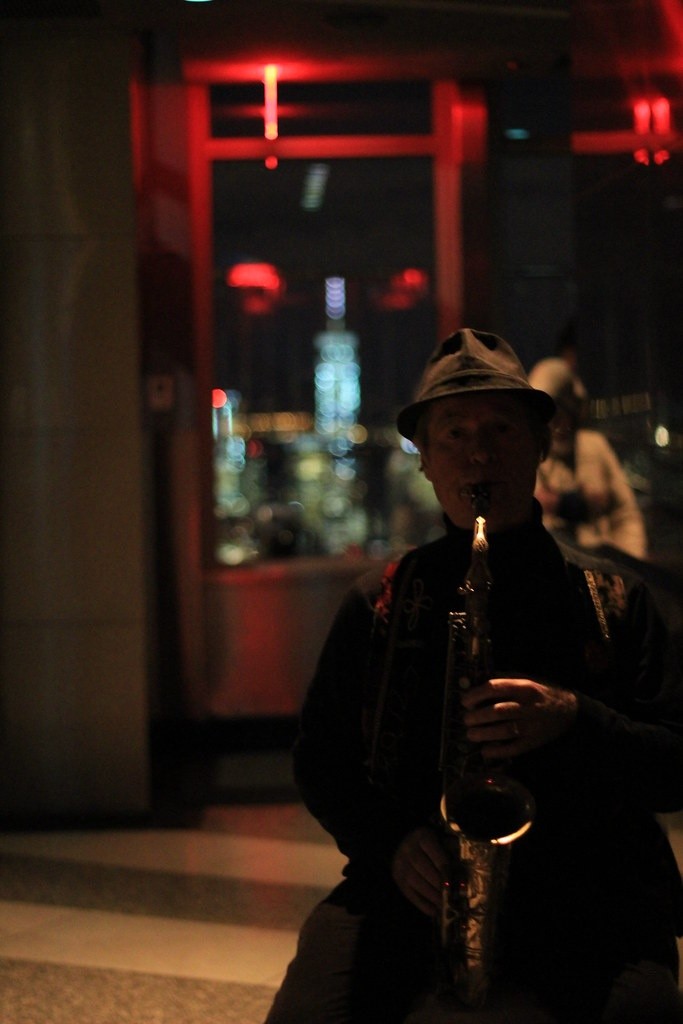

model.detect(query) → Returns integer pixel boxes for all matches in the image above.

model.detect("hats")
[396,329,561,429]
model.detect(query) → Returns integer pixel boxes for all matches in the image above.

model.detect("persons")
[255,330,683,1024]
[516,357,652,567]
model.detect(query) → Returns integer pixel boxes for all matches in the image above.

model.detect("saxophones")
[428,485,539,1007]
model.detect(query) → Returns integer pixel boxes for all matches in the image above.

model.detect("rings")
[510,719,522,738]
